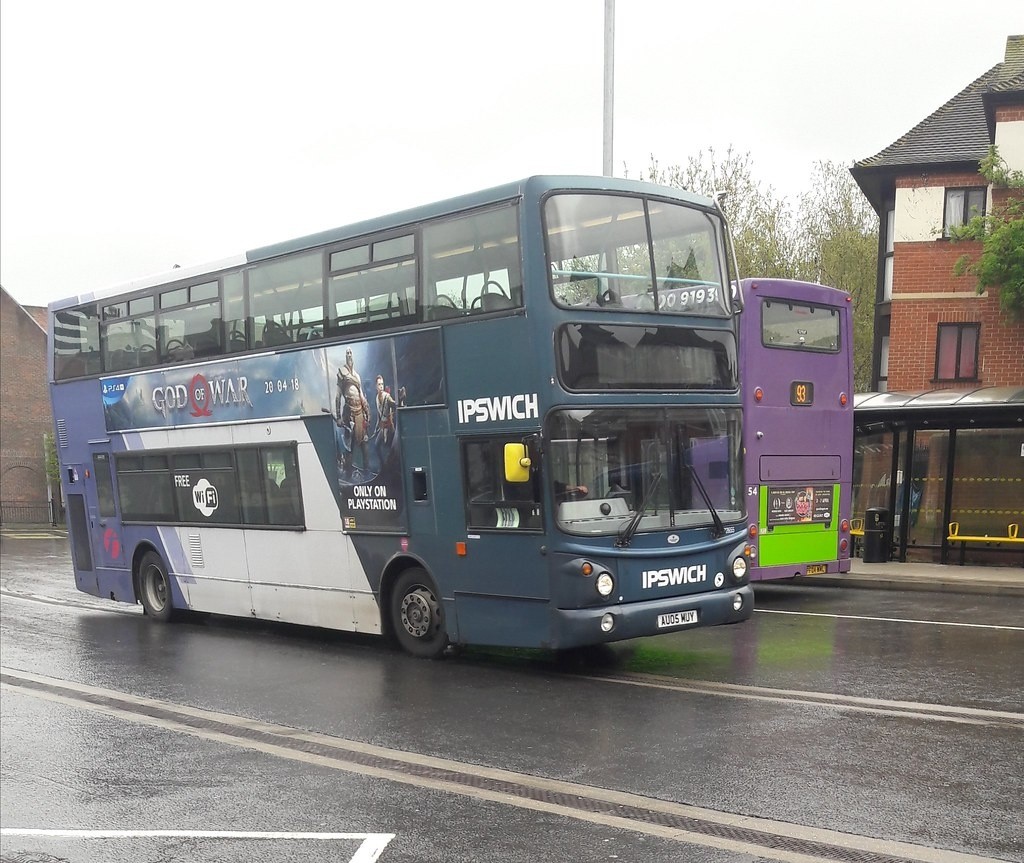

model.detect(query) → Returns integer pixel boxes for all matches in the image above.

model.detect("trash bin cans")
[863,507,890,564]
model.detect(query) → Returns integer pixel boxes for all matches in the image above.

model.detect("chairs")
[597,289,660,310]
[54,281,519,379]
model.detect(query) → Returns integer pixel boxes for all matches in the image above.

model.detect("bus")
[46,176,756,658]
[566,277,855,580]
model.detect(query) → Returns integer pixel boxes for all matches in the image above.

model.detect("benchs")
[850,518,865,559]
[946,522,1024,566]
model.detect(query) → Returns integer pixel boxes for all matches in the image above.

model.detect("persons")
[371,375,406,469]
[335,347,373,483]
[502,452,589,528]
